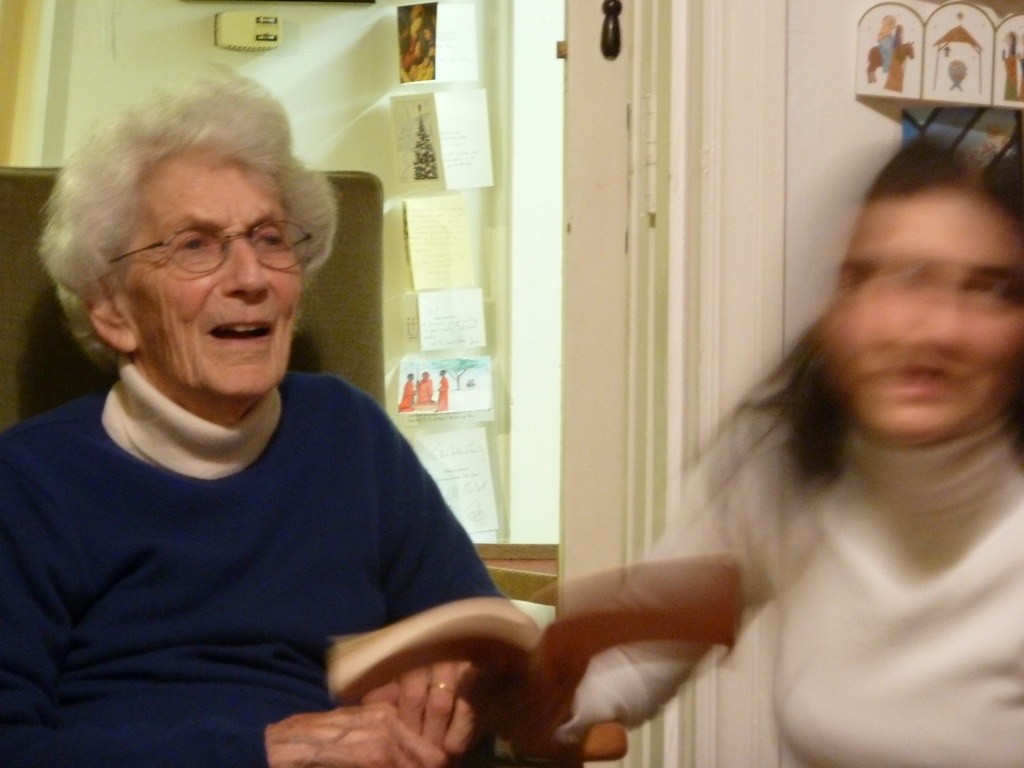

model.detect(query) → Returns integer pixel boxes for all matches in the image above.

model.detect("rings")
[430,683,452,695]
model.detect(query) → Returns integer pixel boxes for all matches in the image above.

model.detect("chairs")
[0,166,389,434]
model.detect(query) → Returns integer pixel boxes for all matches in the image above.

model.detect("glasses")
[108,220,312,276]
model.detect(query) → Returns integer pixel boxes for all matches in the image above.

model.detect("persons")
[508,140,1023,767]
[399,6,435,81]
[0,88,500,768]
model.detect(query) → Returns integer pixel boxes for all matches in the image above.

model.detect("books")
[327,595,559,703]
